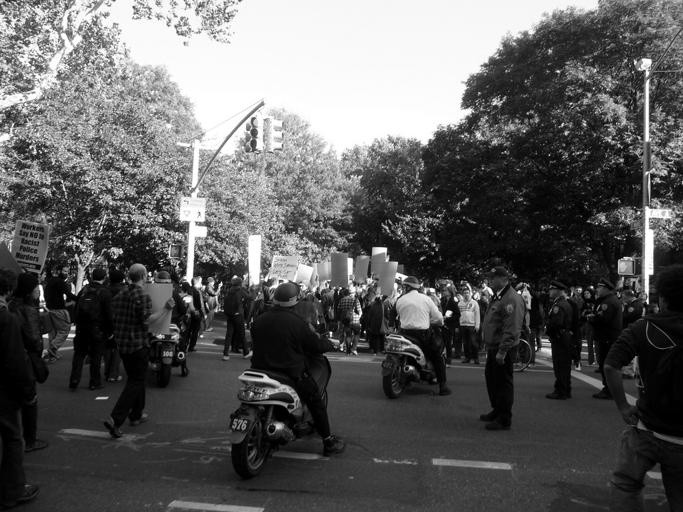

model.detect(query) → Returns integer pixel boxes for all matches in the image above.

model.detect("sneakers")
[104,418,123,437]
[25,439,48,452]
[480,412,498,422]
[48,345,57,356]
[593,391,612,399]
[69,376,123,391]
[546,390,568,400]
[486,419,509,430]
[339,344,357,356]
[223,347,253,360]
[129,413,150,426]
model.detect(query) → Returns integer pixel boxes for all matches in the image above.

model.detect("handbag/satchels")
[380,317,396,335]
[346,310,361,332]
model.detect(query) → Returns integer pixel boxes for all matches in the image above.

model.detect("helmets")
[402,276,421,288]
[272,282,304,307]
[155,271,172,284]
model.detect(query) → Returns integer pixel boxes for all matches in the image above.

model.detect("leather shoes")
[440,385,452,396]
[324,436,344,457]
[446,351,480,364]
[4,484,40,508]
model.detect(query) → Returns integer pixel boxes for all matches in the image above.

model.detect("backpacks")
[223,288,239,316]
[78,287,104,322]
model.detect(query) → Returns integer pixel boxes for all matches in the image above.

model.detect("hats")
[484,266,508,276]
[547,281,566,291]
[597,278,614,290]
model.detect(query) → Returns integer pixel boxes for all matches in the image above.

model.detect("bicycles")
[512,328,533,372]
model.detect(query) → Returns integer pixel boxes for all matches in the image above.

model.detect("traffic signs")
[649,209,675,219]
[181,197,205,222]
[193,225,208,237]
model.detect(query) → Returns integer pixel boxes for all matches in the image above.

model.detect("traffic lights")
[272,118,286,152]
[616,258,636,276]
[249,115,265,152]
[246,121,252,153]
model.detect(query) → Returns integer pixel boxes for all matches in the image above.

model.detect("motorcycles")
[148,322,185,387]
[380,315,454,400]
[230,330,340,479]
